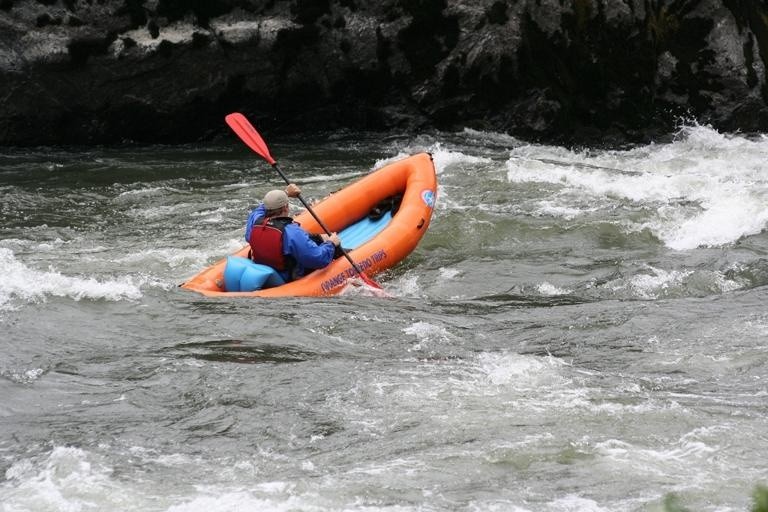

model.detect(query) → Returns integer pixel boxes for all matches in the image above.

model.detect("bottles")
[262,190,289,210]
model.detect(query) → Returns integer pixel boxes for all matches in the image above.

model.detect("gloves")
[177,151,437,299]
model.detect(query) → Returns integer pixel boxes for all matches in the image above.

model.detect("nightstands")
[226,112,386,296]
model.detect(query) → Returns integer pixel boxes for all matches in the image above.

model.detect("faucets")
[391,193,403,219]
[368,197,392,221]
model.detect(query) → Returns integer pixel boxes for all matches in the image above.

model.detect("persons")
[244,182,341,281]
